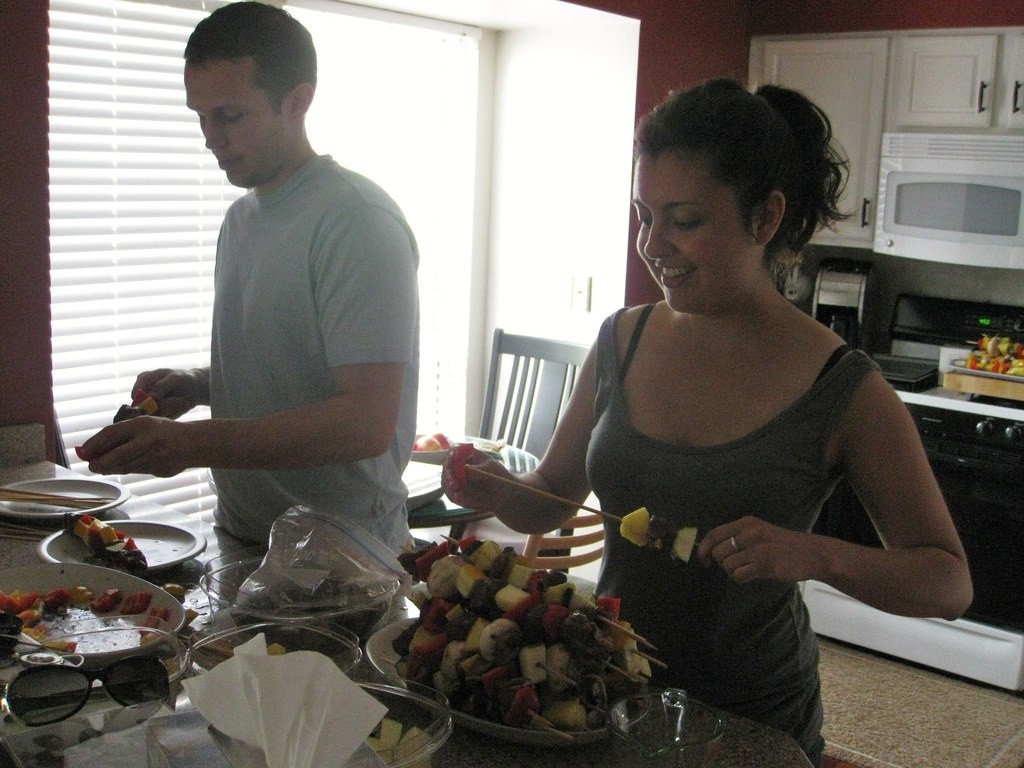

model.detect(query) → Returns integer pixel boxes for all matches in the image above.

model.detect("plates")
[0,478,131,519]
[0,562,186,661]
[364,618,652,743]
[36,519,207,576]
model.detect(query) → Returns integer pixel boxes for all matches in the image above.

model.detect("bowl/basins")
[184,610,363,680]
[198,542,401,645]
[342,676,455,768]
[411,432,458,464]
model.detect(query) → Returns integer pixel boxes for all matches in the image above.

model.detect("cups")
[604,691,727,768]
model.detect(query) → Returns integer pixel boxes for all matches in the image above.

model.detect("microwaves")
[873,131,1024,269]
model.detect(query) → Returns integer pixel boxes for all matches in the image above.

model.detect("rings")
[730,537,740,554]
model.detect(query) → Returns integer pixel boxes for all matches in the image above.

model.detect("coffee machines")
[803,256,883,352]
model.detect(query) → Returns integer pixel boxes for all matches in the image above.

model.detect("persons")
[438,76,974,768]
[74,0,421,559]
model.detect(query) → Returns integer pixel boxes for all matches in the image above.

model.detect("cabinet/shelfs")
[890,26,1024,136]
[747,30,898,250]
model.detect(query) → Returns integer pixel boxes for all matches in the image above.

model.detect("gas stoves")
[875,292,1024,451]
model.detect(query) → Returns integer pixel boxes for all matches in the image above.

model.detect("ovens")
[839,431,1024,635]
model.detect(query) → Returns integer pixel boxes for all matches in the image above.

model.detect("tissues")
[145,630,389,766]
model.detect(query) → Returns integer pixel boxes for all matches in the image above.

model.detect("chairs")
[522,514,607,600]
[479,328,592,574]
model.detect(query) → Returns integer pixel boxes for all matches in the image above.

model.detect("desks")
[401,434,540,552]
